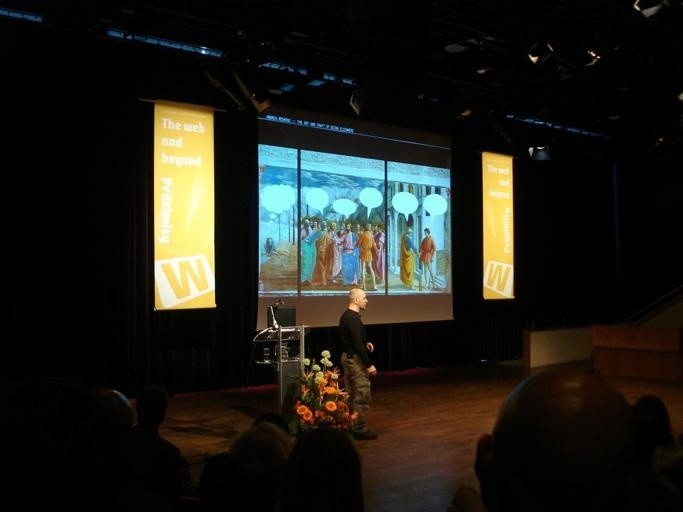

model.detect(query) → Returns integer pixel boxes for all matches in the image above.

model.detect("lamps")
[217,34,275,117]
[525,32,604,70]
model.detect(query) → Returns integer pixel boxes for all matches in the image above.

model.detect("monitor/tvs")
[267,306,296,328]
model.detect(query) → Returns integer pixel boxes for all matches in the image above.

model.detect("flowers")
[290,350,361,425]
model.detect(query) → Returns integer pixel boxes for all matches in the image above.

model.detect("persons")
[300,219,437,289]
[339,288,378,440]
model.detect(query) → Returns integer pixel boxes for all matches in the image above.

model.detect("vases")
[297,416,349,436]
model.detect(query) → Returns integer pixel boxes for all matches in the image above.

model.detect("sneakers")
[353,429,378,441]
